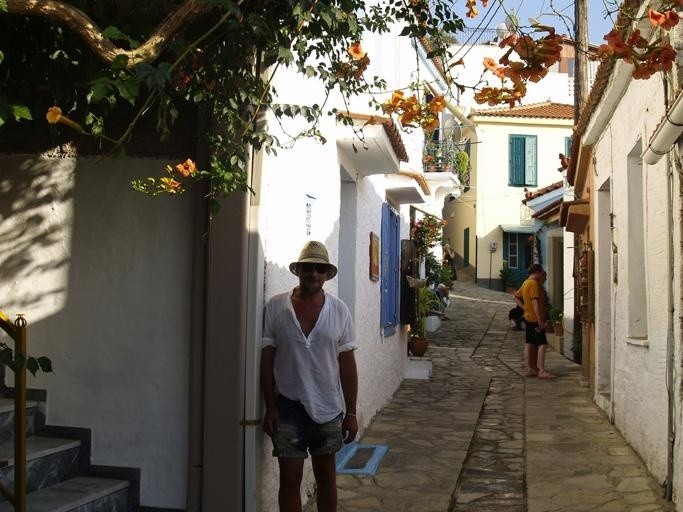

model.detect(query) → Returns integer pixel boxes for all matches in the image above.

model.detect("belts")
[276,394,308,409]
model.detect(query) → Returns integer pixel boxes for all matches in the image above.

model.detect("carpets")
[334,441,389,476]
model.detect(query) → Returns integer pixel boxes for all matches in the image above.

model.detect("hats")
[289,241,338,282]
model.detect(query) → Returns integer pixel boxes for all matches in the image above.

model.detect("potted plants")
[569,333,580,364]
[437,263,453,297]
[406,287,443,357]
[498,260,520,293]
[548,306,565,337]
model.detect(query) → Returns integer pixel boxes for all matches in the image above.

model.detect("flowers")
[438,138,454,163]
[407,211,449,264]
[421,143,441,165]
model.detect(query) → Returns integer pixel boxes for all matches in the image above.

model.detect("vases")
[426,164,437,171]
[439,161,452,171]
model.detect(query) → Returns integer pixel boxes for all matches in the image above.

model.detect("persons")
[433,282,450,321]
[439,242,458,283]
[533,271,552,369]
[508,298,525,329]
[511,263,556,379]
[261,238,361,511]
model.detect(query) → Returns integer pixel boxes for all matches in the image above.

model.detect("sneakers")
[511,325,522,331]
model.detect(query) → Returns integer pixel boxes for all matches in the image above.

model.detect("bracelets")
[344,412,354,419]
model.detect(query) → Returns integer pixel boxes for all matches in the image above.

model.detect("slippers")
[525,370,539,376]
[537,371,558,379]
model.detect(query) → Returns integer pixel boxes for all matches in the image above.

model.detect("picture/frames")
[368,231,379,283]
